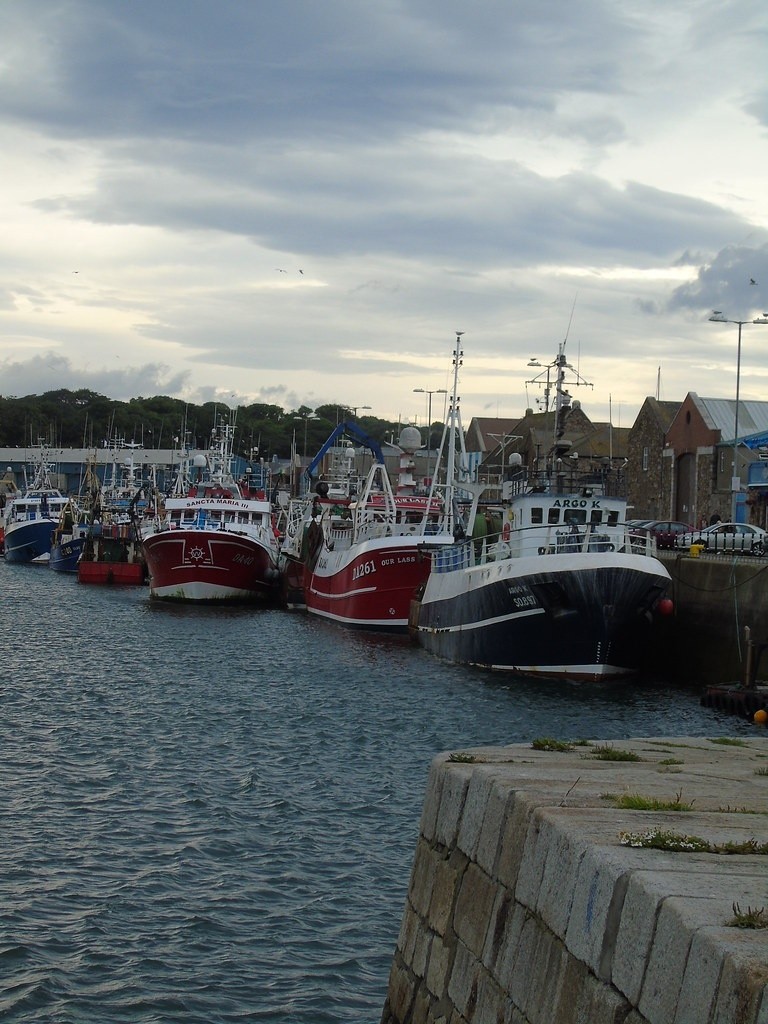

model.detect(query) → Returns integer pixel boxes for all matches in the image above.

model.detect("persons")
[700,510,732,529]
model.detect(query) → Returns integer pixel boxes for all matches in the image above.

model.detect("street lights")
[709,315,768,522]
[413,388,448,487]
[293,416,320,466]
[342,405,372,447]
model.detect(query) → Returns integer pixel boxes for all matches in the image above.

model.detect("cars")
[676,522,768,557]
[625,520,701,548]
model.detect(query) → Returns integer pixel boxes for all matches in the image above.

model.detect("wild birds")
[749,278,759,287]
[72,270,81,274]
[275,268,289,273]
[299,269,305,275]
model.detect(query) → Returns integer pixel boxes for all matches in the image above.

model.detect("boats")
[131,392,283,604]
[272,409,419,633]
[49,410,94,573]
[4,421,71,562]
[75,408,148,584]
[410,291,673,691]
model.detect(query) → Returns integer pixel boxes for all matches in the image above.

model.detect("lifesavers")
[503,523,510,541]
